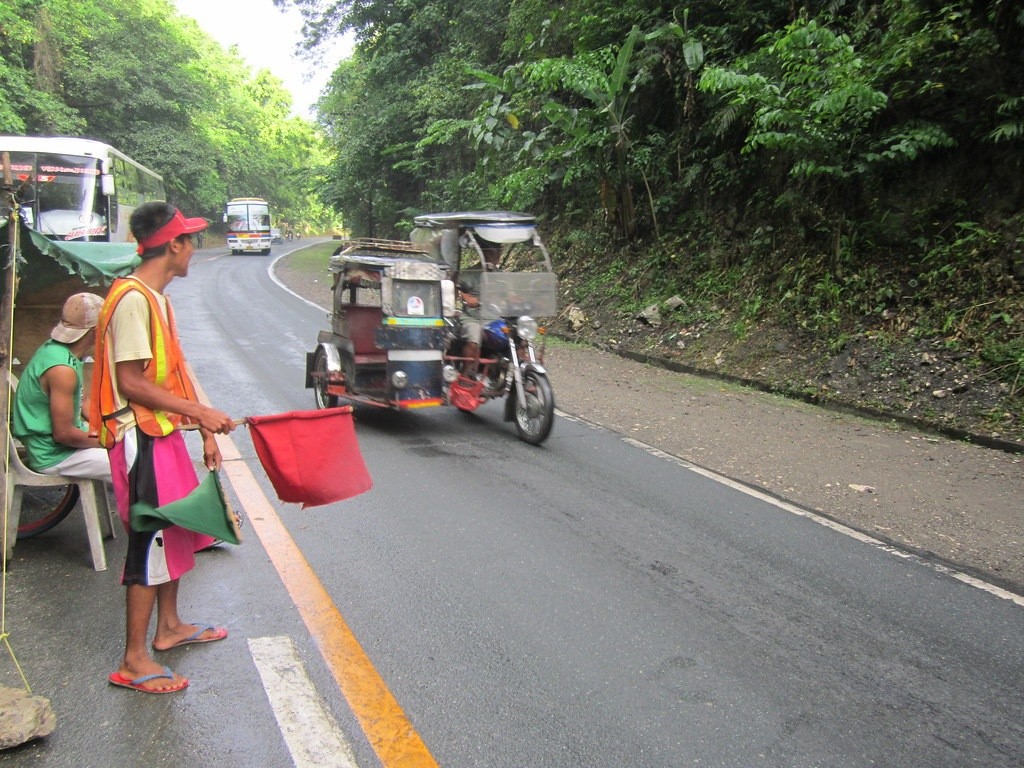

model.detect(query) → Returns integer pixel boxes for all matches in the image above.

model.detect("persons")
[196,232,203,248]
[453,238,531,380]
[12,293,243,547]
[88,201,238,695]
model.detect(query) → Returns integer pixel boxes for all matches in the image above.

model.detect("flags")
[128,471,241,545]
[247,407,373,509]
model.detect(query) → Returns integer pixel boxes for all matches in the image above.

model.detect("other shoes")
[462,380,488,391]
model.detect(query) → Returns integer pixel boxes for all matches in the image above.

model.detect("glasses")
[483,249,502,253]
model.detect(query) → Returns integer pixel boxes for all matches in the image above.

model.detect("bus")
[1,136,166,245]
[222,197,271,254]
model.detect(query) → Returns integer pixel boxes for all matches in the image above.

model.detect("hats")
[483,240,503,248]
[136,206,210,256]
[287,227,291,230]
[49,292,106,342]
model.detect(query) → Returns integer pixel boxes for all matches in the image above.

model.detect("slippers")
[109,664,189,693]
[153,621,227,650]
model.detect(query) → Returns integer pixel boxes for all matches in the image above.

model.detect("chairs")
[2,366,117,572]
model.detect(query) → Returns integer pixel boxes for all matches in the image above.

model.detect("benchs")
[340,305,388,369]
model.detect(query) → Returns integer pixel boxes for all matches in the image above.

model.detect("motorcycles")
[305,212,555,445]
[285,232,300,242]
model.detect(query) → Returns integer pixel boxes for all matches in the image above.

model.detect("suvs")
[271,230,284,244]
